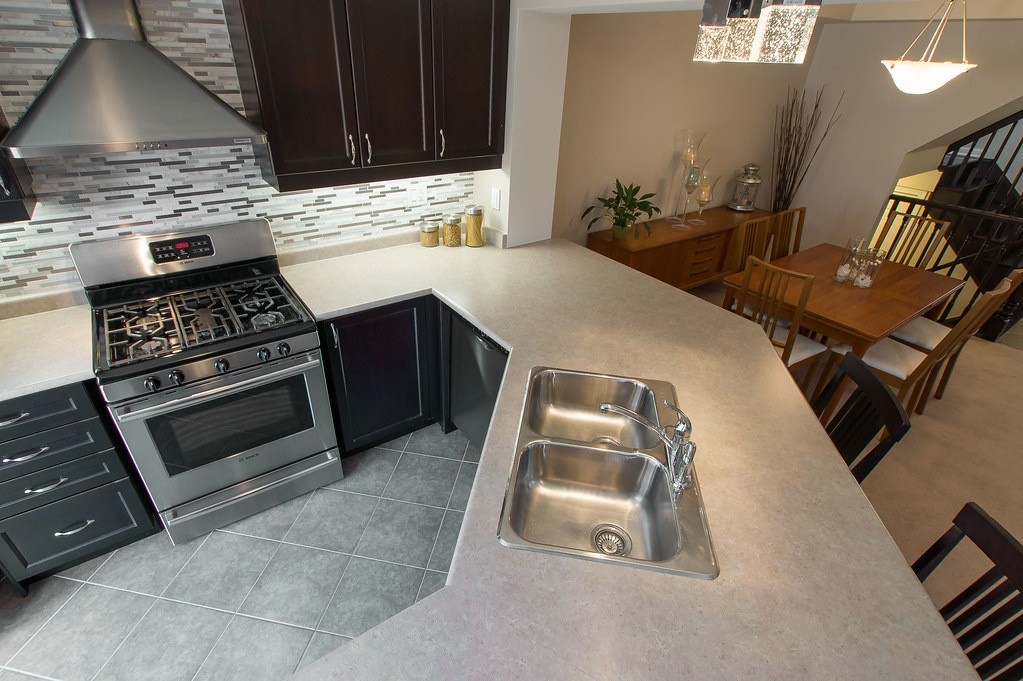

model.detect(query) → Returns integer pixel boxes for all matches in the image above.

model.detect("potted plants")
[581,177,661,241]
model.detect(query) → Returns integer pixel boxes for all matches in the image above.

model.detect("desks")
[723,242,966,406]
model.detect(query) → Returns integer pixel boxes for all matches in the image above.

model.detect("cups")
[833,233,886,289]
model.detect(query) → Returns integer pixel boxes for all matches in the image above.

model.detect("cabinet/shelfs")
[315,291,508,456]
[0,379,163,599]
[587,209,775,290]
[223,0,514,193]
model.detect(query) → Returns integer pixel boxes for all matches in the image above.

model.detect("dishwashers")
[449,311,509,454]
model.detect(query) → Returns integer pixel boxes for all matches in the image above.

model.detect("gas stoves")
[68,218,320,404]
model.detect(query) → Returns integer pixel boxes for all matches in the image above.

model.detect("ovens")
[103,348,344,547]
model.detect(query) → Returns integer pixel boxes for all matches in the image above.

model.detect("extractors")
[0,0,268,159]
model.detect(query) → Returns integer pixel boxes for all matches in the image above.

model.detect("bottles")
[464,204,485,247]
[419,221,439,248]
[441,214,462,247]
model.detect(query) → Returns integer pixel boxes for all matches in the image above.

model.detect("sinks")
[519,366,690,453]
[497,439,721,581]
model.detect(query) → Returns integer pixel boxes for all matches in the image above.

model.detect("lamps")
[881,0,978,95]
[692,0,823,65]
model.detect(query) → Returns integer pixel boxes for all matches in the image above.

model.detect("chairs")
[909,500,1023,680]
[727,205,1022,484]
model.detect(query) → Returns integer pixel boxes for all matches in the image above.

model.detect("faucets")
[598,399,697,491]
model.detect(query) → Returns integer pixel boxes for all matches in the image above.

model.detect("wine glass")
[665,126,722,232]
